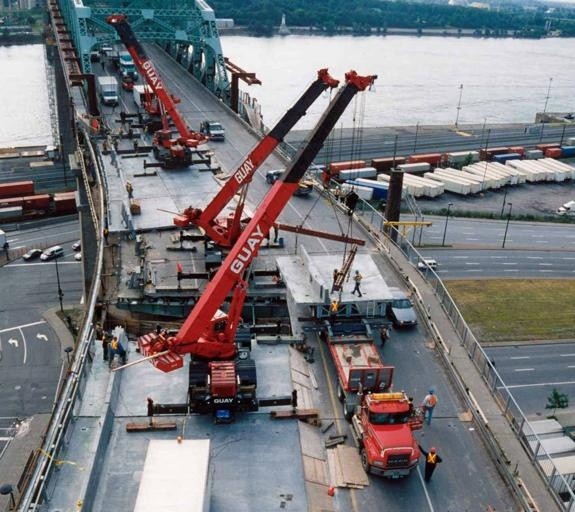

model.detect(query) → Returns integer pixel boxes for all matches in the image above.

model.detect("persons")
[95,321,110,360]
[125,179,134,201]
[350,269,363,297]
[330,268,345,294]
[102,125,149,151]
[380,329,390,348]
[3,243,10,260]
[100,60,107,70]
[417,443,443,482]
[421,387,439,425]
[333,185,340,200]
[340,187,347,203]
[108,335,127,368]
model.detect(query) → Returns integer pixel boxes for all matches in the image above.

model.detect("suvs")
[203,121,226,142]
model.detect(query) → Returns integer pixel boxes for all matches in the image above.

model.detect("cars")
[115,50,139,91]
[22,239,82,261]
[418,260,437,270]
[386,291,419,329]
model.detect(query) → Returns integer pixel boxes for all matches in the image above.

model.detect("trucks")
[132,85,156,108]
[558,201,575,216]
[97,76,118,107]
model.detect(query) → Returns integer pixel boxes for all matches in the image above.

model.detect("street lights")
[441,203,453,247]
[501,180,512,247]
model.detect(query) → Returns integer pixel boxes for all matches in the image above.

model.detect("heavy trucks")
[309,305,420,479]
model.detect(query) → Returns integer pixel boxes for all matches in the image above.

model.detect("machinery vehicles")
[172,68,340,287]
[108,16,210,168]
[109,70,379,424]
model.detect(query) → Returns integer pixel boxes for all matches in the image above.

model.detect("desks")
[501,180,512,247]
[441,203,453,247]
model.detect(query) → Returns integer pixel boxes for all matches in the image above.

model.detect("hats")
[418,260,437,270]
[22,239,82,261]
